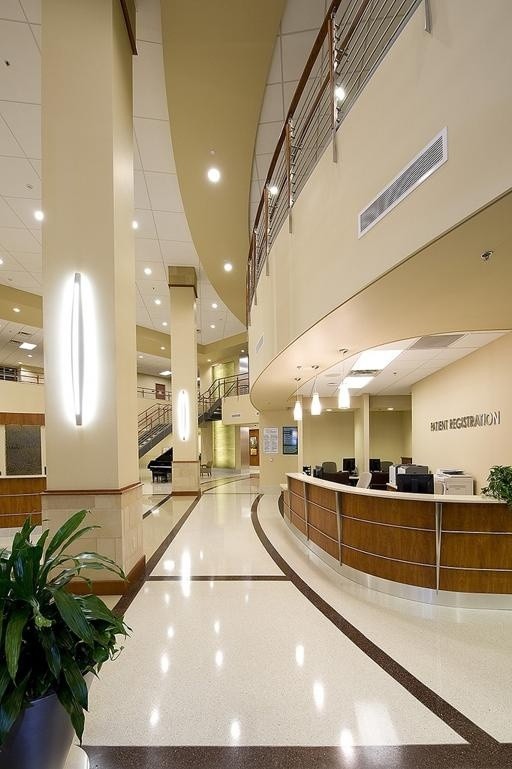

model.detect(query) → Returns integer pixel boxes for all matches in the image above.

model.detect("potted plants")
[0,508,134,769]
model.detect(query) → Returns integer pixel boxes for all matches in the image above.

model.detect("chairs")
[200,460,214,477]
[354,471,372,489]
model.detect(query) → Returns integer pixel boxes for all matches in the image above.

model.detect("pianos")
[147,448,172,482]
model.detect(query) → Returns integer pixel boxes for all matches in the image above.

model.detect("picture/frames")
[282,426,298,454]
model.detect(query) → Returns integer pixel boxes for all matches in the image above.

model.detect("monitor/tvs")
[342,458,356,474]
[369,458,382,473]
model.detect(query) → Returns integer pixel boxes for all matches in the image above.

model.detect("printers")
[433,467,474,495]
